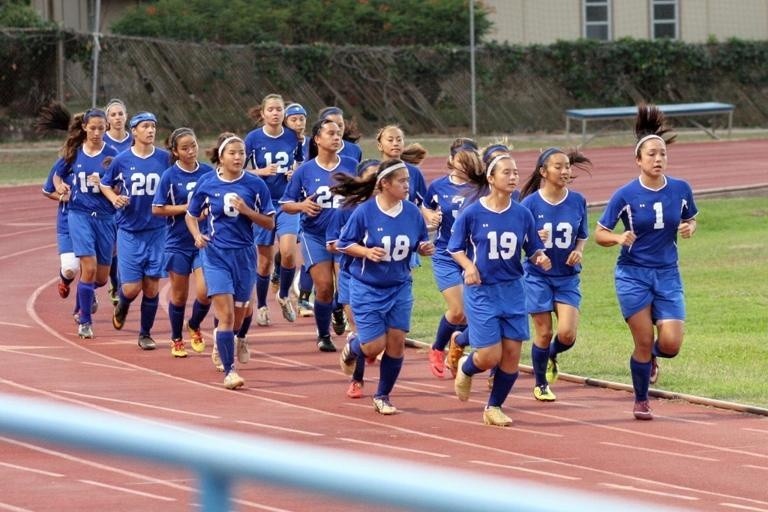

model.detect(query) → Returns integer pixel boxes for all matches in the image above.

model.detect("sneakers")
[277,292,296,322]
[633,398,653,420]
[138,320,251,390]
[298,299,315,317]
[428,331,562,426]
[315,306,398,415]
[256,307,271,326]
[58,279,123,339]
[649,353,659,384]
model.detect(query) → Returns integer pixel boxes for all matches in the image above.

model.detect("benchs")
[565,101,735,150]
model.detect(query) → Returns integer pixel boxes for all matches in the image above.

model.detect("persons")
[43,97,276,390]
[243,96,437,415]
[421,137,589,426]
[595,103,699,420]
